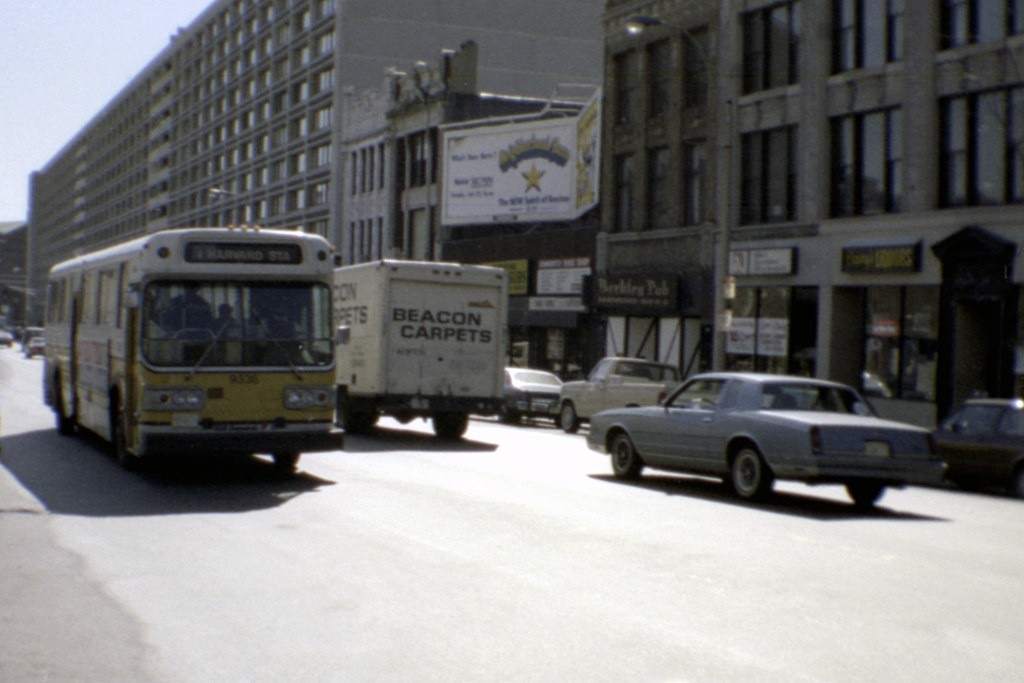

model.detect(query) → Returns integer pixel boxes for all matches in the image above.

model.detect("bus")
[44,223,337,477]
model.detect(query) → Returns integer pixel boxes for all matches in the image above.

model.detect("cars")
[0,324,47,358]
[587,370,932,512]
[503,365,563,425]
[924,396,1023,500]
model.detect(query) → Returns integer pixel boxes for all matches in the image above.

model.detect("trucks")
[328,258,505,437]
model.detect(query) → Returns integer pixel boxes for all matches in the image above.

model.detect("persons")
[248,294,301,350]
[167,277,215,348]
[213,301,244,344]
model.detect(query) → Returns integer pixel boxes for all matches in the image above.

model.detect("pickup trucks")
[554,355,725,432]
